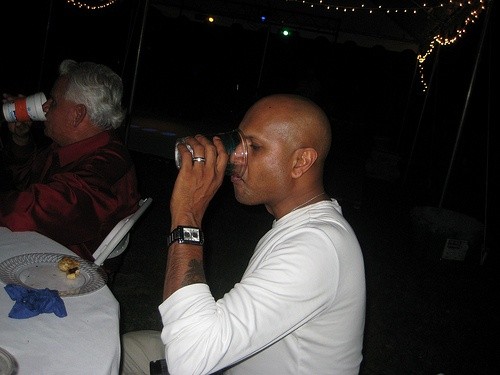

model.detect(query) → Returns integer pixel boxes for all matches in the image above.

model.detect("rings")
[192,157,206,162]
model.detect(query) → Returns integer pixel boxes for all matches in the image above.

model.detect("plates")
[0,348,19,375]
[0,253,109,296]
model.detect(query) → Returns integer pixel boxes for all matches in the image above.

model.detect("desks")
[0,225,122,375]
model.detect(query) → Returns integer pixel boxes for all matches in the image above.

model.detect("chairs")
[94,194,155,271]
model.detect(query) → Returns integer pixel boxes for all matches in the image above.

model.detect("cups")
[3,92,47,122]
[176,128,248,178]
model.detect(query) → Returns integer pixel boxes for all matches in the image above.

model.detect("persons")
[121,92,366,375]
[0,60,141,276]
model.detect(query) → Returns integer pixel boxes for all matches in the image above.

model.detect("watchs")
[166,225,207,249]
